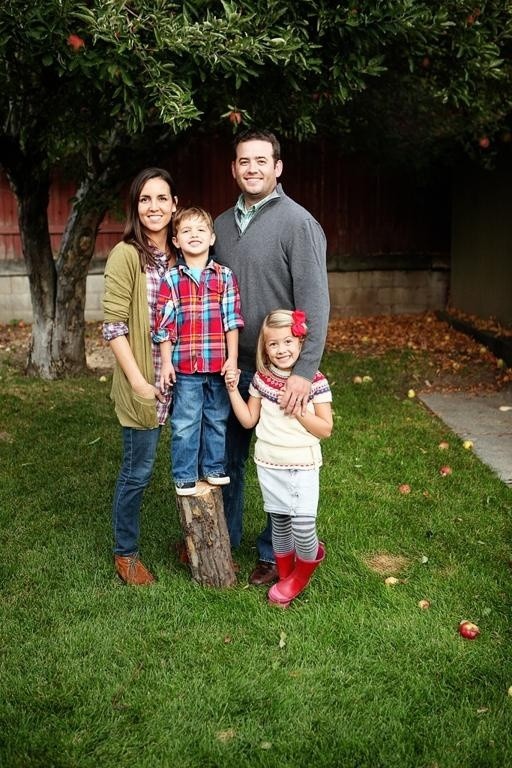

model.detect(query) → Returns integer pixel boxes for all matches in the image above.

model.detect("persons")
[211,128,331,588]
[148,200,242,495]
[100,166,181,589]
[222,309,334,605]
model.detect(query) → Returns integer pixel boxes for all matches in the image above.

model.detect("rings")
[296,398,303,402]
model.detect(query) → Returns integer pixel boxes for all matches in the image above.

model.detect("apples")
[418,600,429,609]
[363,375,373,384]
[408,388,416,398]
[459,620,479,639]
[441,465,452,477]
[230,112,241,125]
[463,440,474,450]
[479,137,490,148]
[69,34,84,53]
[439,440,449,450]
[353,376,362,384]
[398,484,410,494]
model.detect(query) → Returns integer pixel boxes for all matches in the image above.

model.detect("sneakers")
[113,552,155,586]
[199,473,231,487]
[173,482,198,498]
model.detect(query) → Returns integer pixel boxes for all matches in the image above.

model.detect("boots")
[267,544,326,601]
[268,548,296,609]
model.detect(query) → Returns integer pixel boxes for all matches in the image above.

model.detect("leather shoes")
[247,560,279,586]
